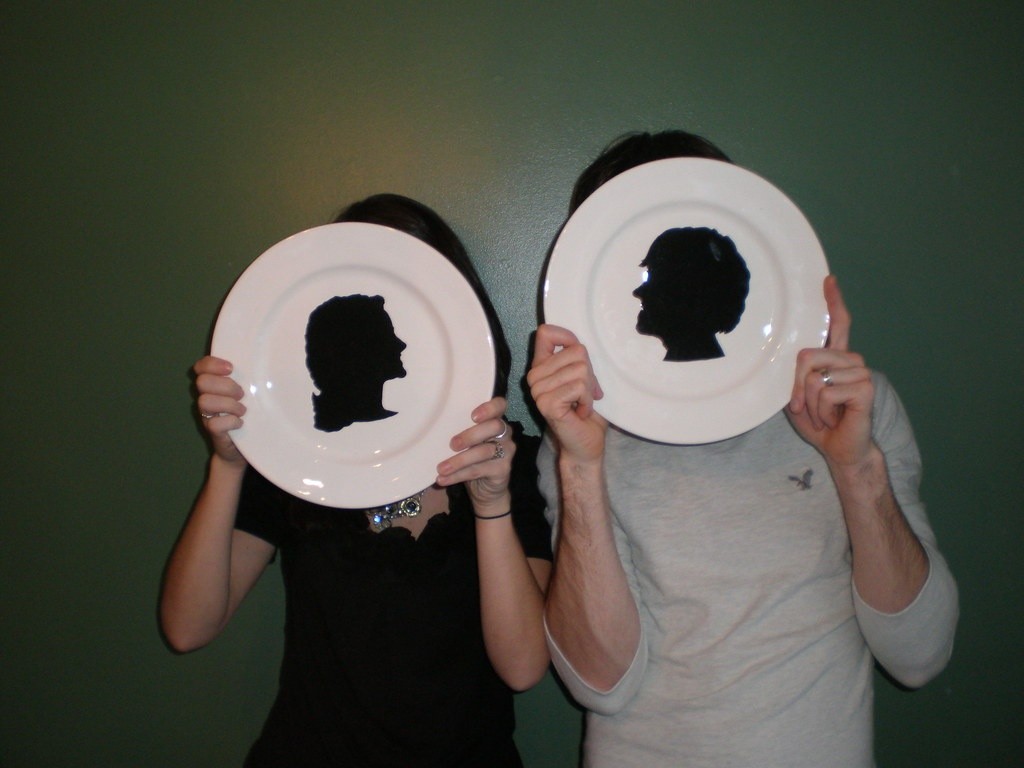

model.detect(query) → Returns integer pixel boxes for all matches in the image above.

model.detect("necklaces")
[364,489,425,529]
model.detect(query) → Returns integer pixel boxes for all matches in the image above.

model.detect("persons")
[158,191,553,768]
[527,126,959,768]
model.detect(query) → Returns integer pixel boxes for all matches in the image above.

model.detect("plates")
[543,157,831,445]
[211,222,496,509]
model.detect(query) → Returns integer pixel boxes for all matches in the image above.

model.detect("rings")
[494,416,507,439]
[200,413,215,418]
[488,440,505,459]
[818,368,834,386]
